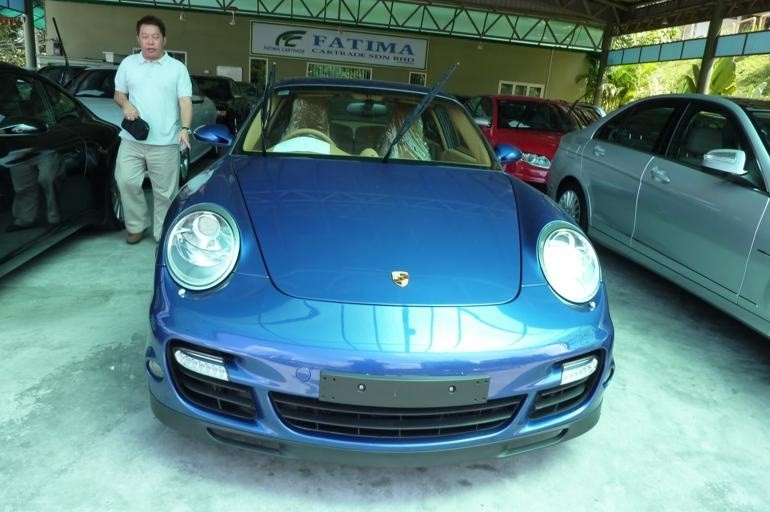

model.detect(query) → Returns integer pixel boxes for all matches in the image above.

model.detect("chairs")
[681,126,726,167]
[282,97,331,138]
[379,108,431,161]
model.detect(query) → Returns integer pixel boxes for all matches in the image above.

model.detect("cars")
[180,74,259,184]
[537,92,770,339]
[544,99,607,135]
[461,94,584,193]
[1,61,123,285]
[1,60,123,285]
[61,66,127,128]
[35,57,101,91]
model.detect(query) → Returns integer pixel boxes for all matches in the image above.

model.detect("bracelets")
[179,126,190,131]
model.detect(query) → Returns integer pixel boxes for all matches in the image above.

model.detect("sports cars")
[141,75,623,475]
[144,75,616,469]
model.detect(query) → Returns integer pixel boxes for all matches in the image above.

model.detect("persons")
[112,14,193,243]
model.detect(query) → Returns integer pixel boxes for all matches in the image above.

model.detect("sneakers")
[8,221,37,231]
[127,229,147,244]
[47,223,59,231]
